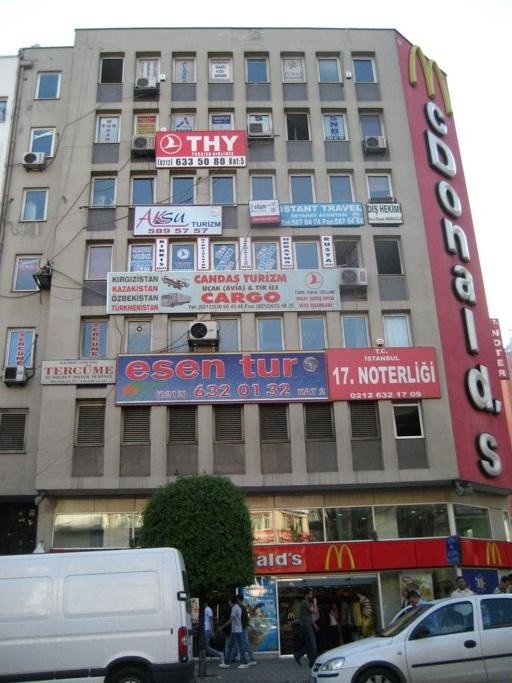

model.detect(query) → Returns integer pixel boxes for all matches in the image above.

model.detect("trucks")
[160,292,192,308]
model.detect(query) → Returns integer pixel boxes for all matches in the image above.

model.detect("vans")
[0,547,193,683]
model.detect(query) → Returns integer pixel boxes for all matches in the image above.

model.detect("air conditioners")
[338,267,369,290]
[3,364,25,387]
[133,76,157,90]
[23,152,44,169]
[131,135,155,155]
[363,135,387,153]
[188,321,219,346]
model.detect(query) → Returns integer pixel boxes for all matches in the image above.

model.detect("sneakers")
[237,662,256,668]
[219,652,230,668]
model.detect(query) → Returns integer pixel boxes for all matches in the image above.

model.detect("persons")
[407,591,423,607]
[193,598,225,664]
[450,577,473,597]
[288,587,375,668]
[506,574,512,594]
[493,576,509,594]
[219,592,257,669]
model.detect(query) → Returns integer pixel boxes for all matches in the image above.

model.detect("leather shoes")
[294,652,301,665]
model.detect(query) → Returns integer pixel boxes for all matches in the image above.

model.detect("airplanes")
[161,275,192,290]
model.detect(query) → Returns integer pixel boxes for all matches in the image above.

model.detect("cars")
[310,593,512,683]
